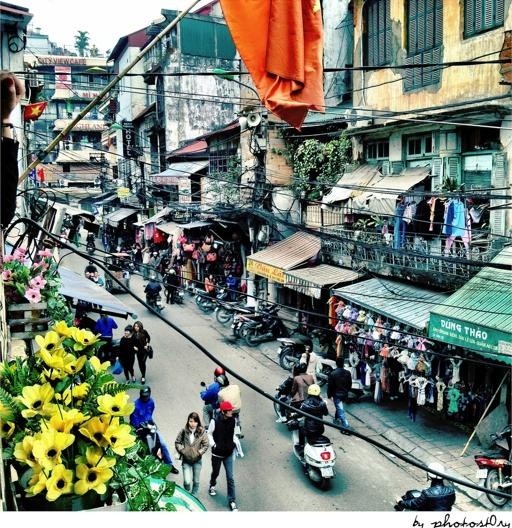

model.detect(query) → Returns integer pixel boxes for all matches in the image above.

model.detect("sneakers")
[209,485,217,495]
[276,416,287,422]
[229,501,238,510]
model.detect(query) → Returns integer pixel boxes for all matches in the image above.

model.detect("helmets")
[299,362,307,372]
[427,461,446,478]
[140,387,150,396]
[307,384,321,396]
[294,363,300,374]
[216,375,229,387]
[214,367,225,376]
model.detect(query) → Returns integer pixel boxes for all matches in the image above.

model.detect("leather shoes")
[141,377,145,384]
[171,465,178,474]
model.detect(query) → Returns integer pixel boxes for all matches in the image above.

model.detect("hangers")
[332,299,462,416]
[397,188,466,213]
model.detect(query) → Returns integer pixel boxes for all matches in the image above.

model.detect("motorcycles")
[160,277,184,304]
[192,287,290,347]
[86,236,97,254]
[275,399,337,492]
[275,325,374,403]
[89,272,100,284]
[472,422,512,508]
[395,489,424,502]
[141,284,165,314]
[272,377,293,423]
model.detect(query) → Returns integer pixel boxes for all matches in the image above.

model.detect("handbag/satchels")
[148,345,153,358]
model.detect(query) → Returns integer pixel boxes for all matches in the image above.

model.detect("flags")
[214,0,326,134]
[25,99,52,123]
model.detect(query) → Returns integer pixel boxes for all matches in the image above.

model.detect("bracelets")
[1,120,16,130]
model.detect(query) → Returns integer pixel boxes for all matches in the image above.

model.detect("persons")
[213,374,244,439]
[206,401,244,511]
[73,311,154,385]
[43,214,239,311]
[273,337,353,456]
[200,366,225,430]
[392,461,458,510]
[130,385,180,473]
[0,67,28,232]
[174,411,209,495]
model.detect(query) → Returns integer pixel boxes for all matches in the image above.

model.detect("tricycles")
[100,252,135,294]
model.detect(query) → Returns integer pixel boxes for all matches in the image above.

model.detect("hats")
[219,400,235,411]
